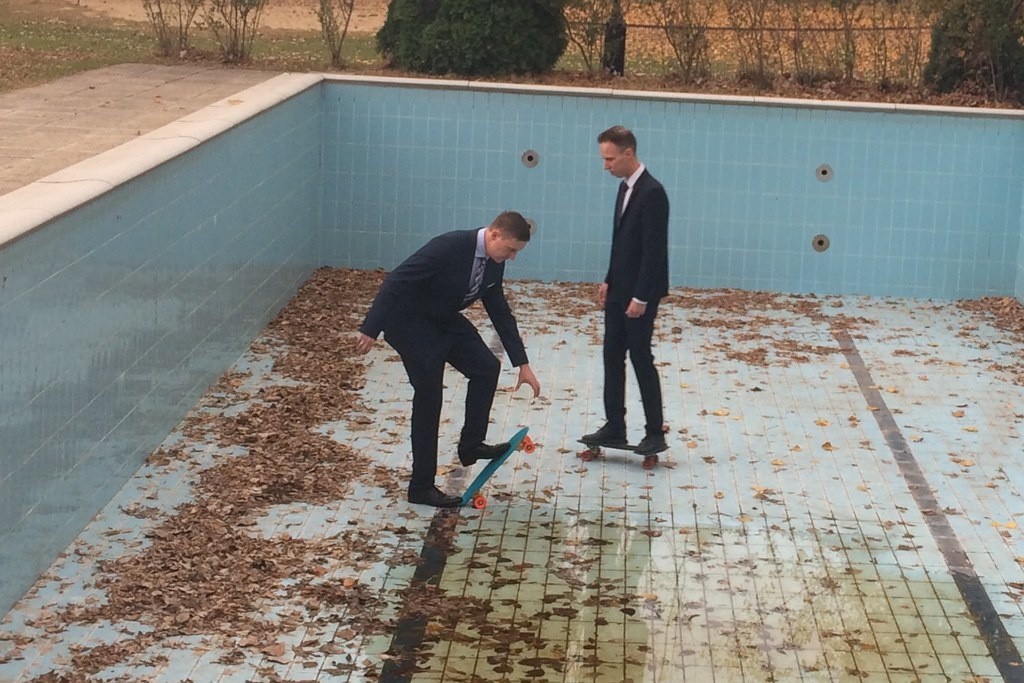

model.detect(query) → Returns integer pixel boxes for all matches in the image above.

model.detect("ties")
[462,257,487,305]
[616,183,629,228]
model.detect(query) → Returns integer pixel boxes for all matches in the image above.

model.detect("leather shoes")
[635,434,666,454]
[582,432,628,445]
[408,486,462,506]
[458,443,510,467]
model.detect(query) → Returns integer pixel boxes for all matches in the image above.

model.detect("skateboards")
[576,440,671,469]
[459,426,534,509]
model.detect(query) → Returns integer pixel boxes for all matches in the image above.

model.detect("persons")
[355,210,543,509]
[579,124,671,455]
